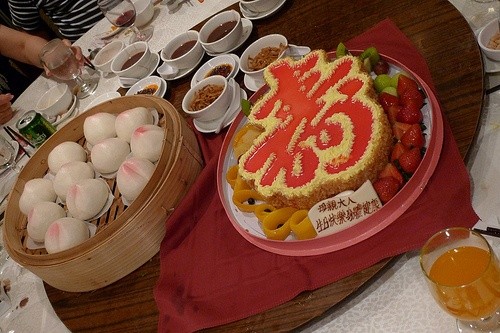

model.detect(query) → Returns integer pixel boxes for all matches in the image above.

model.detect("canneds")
[16,109,57,150]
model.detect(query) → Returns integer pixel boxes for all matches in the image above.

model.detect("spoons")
[117,75,139,86]
[239,2,259,17]
[215,78,241,134]
[157,61,179,78]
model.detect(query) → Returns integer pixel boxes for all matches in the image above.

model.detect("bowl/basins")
[125,76,161,96]
[92,40,154,79]
[160,0,289,122]
[133,0,155,27]
[478,17,500,62]
[36,83,73,117]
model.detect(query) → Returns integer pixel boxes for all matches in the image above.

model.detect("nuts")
[487,33,500,51]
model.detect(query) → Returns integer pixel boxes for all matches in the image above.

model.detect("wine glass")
[0,134,22,176]
[38,38,97,99]
[419,227,500,333]
[97,0,154,45]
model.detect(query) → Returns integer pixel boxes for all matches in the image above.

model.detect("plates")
[164,0,303,133]
[42,94,77,126]
[84,76,167,111]
[96,18,121,39]
[0,141,19,174]
[472,27,500,73]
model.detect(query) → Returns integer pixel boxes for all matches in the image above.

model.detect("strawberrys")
[371,74,425,204]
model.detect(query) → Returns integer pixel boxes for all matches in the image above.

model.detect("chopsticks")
[3,126,35,158]
[473,226,500,238]
[83,56,95,71]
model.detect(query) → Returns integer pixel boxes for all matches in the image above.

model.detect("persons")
[8,0,124,45]
[0,24,84,127]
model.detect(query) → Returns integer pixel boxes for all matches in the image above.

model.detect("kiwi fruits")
[336,43,379,69]
[240,98,255,117]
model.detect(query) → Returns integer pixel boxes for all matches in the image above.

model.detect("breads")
[235,47,391,207]
